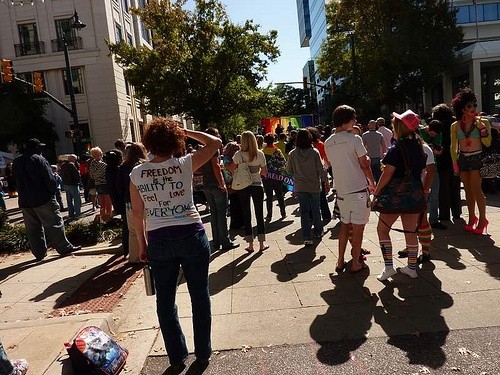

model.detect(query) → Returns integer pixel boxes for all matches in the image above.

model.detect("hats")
[26,138,46,148]
[392,109,421,131]
[375,117,385,124]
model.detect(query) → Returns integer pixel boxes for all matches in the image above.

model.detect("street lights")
[275,81,332,91]
[62,7,88,156]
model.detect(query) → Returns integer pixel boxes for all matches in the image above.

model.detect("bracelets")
[478,124,489,137]
[184,128,188,136]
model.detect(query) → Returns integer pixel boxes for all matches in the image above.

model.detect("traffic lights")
[31,70,45,92]
[0,58,15,83]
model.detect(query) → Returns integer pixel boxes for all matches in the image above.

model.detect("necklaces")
[460,120,478,137]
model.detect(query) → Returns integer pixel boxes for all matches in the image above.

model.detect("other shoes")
[417,252,431,264]
[351,248,370,260]
[397,265,417,279]
[67,245,82,253]
[223,242,241,250]
[304,230,324,244]
[431,222,448,230]
[398,248,418,256]
[376,268,396,281]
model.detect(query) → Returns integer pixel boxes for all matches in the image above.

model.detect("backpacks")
[65,326,129,375]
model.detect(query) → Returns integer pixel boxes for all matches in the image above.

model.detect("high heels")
[464,216,489,235]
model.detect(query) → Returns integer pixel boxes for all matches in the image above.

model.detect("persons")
[0,105,455,375]
[451,89,492,235]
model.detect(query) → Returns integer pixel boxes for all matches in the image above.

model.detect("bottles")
[143,262,155,296]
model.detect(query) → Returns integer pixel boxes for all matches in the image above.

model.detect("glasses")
[467,104,477,108]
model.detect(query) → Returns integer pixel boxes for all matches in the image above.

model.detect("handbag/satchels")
[370,141,428,233]
[480,141,500,178]
[231,150,254,190]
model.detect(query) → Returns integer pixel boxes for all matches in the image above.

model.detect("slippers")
[335,261,368,273]
[259,245,269,251]
[245,247,254,253]
